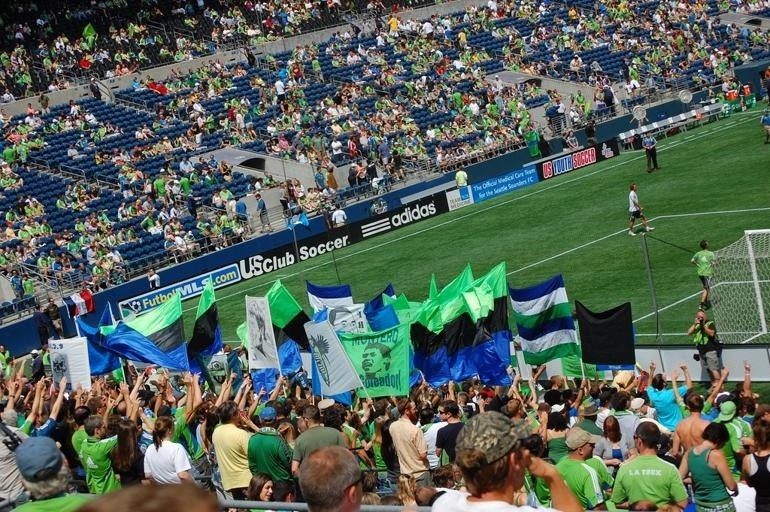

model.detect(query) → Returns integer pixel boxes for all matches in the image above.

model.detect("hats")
[564,429,601,449]
[454,412,528,468]
[14,436,63,481]
[580,402,602,418]
[318,398,335,411]
[259,407,278,424]
[630,398,650,409]
[30,349,39,355]
[714,391,739,421]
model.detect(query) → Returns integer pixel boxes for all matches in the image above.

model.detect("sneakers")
[628,230,636,236]
[646,227,655,232]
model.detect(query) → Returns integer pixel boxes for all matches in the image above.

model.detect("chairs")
[1,61,256,294]
[429,2,769,151]
[114,37,552,205]
[1,1,433,98]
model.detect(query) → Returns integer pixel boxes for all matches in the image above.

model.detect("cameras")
[694,354,699,361]
[699,318,704,325]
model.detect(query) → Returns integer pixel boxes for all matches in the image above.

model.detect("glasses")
[633,436,641,440]
[402,398,411,412]
[438,410,449,414]
